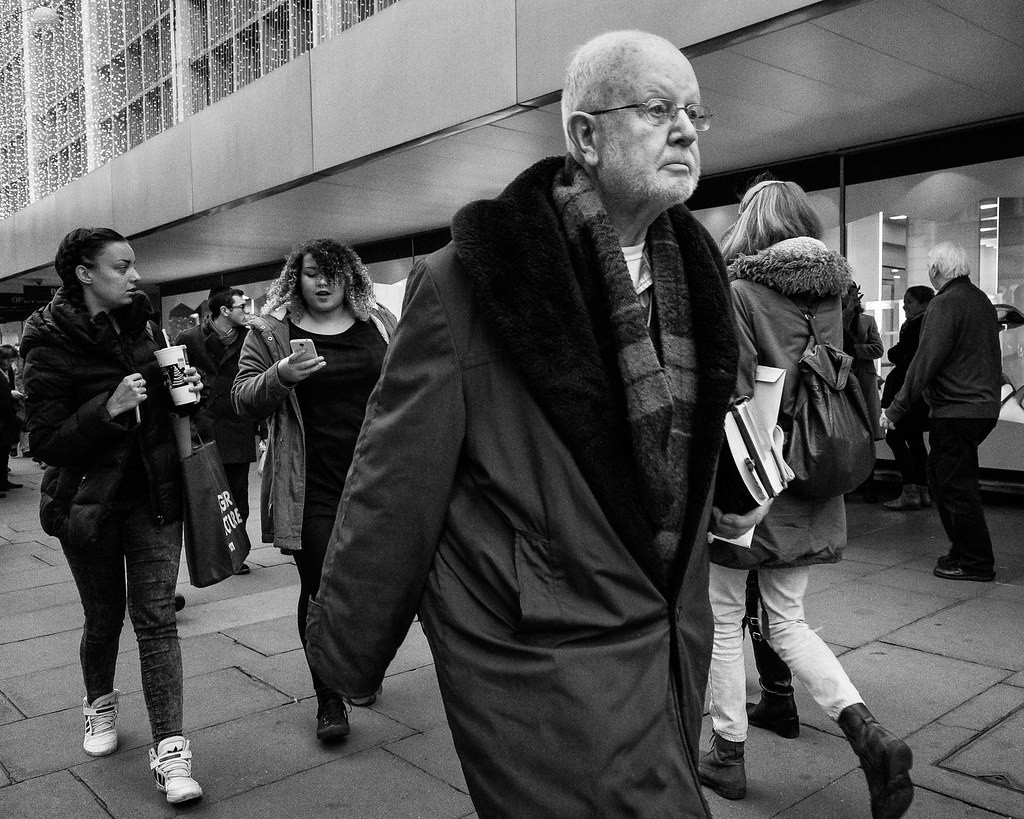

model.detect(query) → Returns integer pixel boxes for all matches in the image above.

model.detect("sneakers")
[316,693,352,735]
[150,735,202,803]
[82,688,121,755]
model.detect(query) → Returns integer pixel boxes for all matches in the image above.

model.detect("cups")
[154,345,197,406]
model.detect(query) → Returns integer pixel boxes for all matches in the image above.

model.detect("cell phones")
[290,338,318,359]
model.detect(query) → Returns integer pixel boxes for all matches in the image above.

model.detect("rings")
[137,380,142,388]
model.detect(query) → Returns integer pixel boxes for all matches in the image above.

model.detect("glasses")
[228,303,247,309]
[585,98,714,132]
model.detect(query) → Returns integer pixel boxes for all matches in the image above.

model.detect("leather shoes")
[937,549,956,566]
[934,561,994,582]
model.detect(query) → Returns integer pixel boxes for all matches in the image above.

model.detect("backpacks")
[784,293,877,497]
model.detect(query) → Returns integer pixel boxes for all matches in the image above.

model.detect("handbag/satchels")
[179,419,251,588]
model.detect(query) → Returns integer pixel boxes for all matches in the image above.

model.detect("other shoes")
[23,451,34,456]
[234,563,250,574]
[9,447,18,457]
[0,480,23,488]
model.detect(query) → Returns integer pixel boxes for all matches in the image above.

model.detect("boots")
[838,703,914,819]
[921,486,932,505]
[698,728,746,799]
[884,484,922,508]
[746,677,799,737]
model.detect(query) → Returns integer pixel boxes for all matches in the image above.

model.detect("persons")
[305,29,738,819]
[699,180,915,818]
[850,285,884,497]
[877,242,1002,581]
[176,290,267,575]
[231,238,397,741]
[0,344,48,498]
[881,286,934,510]
[21,228,204,804]
[742,569,799,738]
